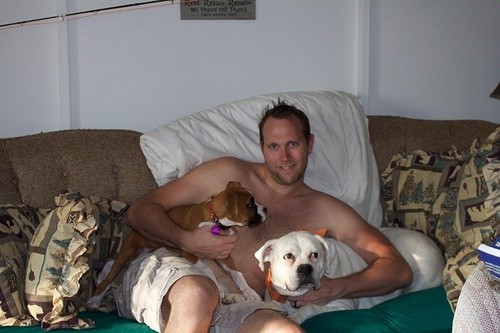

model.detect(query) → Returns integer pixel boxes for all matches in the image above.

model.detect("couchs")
[1,115,499,332]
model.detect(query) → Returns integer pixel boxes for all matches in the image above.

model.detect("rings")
[294,299,298,308]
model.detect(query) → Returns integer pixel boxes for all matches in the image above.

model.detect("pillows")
[23,187,132,332]
[379,125,499,313]
[0,203,41,327]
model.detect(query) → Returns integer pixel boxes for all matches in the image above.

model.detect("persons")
[108,96,414,333]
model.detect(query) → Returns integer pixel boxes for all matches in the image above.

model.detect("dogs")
[91,180,268,306]
[254,226,445,326]
